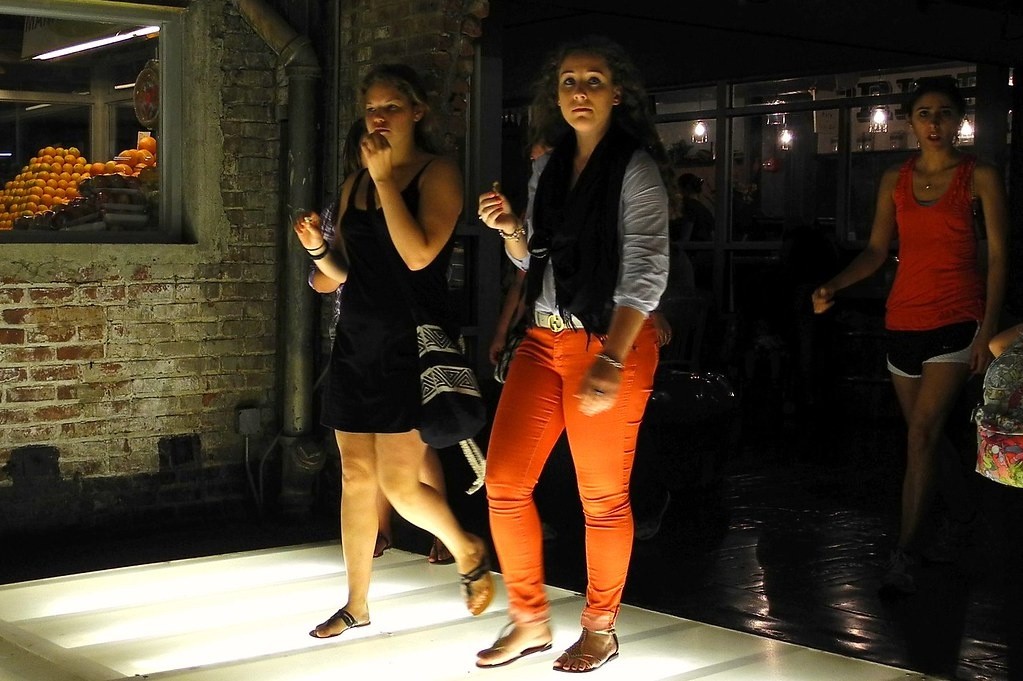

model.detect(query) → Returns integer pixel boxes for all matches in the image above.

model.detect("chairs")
[656,291,707,491]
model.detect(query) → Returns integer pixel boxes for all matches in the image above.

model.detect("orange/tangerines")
[0,136,157,230]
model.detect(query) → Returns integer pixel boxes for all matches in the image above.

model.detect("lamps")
[957,115,975,143]
[691,87,711,145]
[869,71,887,134]
[765,86,793,151]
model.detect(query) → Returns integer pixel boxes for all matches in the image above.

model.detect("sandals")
[552,626,620,672]
[477,620,553,668]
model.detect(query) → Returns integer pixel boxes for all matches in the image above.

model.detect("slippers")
[308,607,371,638]
[457,552,491,616]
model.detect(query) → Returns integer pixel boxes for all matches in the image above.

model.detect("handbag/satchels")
[415,323,487,447]
[973,336,1022,487]
[493,308,532,383]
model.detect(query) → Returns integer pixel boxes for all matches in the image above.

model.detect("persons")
[293,63,495,639]
[988,324,1023,357]
[813,79,1006,570]
[308,119,456,565]
[488,134,676,539]
[475,33,669,673]
[677,173,716,361]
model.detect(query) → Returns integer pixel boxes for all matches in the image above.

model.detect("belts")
[533,311,584,332]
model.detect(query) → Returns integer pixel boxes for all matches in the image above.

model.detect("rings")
[594,389,604,396]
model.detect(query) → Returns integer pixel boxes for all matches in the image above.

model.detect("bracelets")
[499,219,526,242]
[304,239,330,260]
[594,352,626,370]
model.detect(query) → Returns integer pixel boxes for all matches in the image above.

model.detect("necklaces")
[918,154,952,192]
